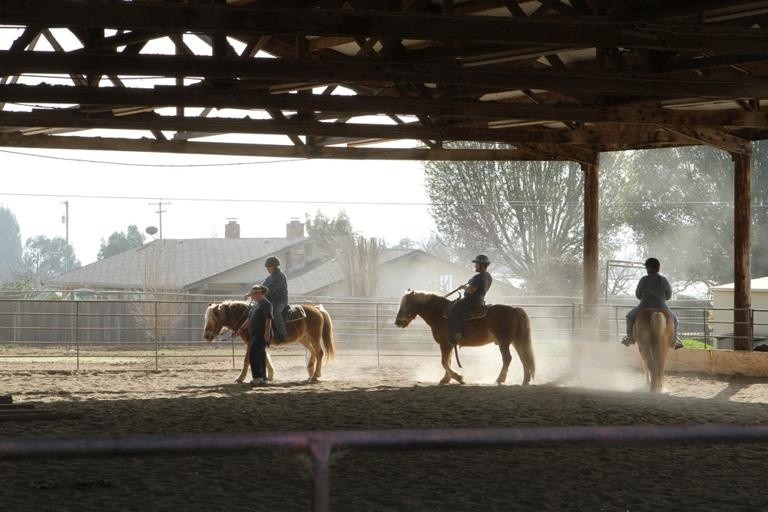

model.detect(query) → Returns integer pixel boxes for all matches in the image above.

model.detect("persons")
[618,258,684,350]
[230,284,273,386]
[444,253,493,346]
[260,256,289,344]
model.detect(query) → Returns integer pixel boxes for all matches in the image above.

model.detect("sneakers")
[620,334,634,347]
[672,337,682,347]
[447,332,465,344]
[275,335,291,345]
[249,377,268,386]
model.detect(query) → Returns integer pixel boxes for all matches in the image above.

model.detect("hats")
[265,256,280,267]
[472,254,492,265]
[643,258,660,268]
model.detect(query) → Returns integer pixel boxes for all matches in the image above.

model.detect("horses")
[202,300,335,384]
[631,308,676,394]
[394,288,536,386]
